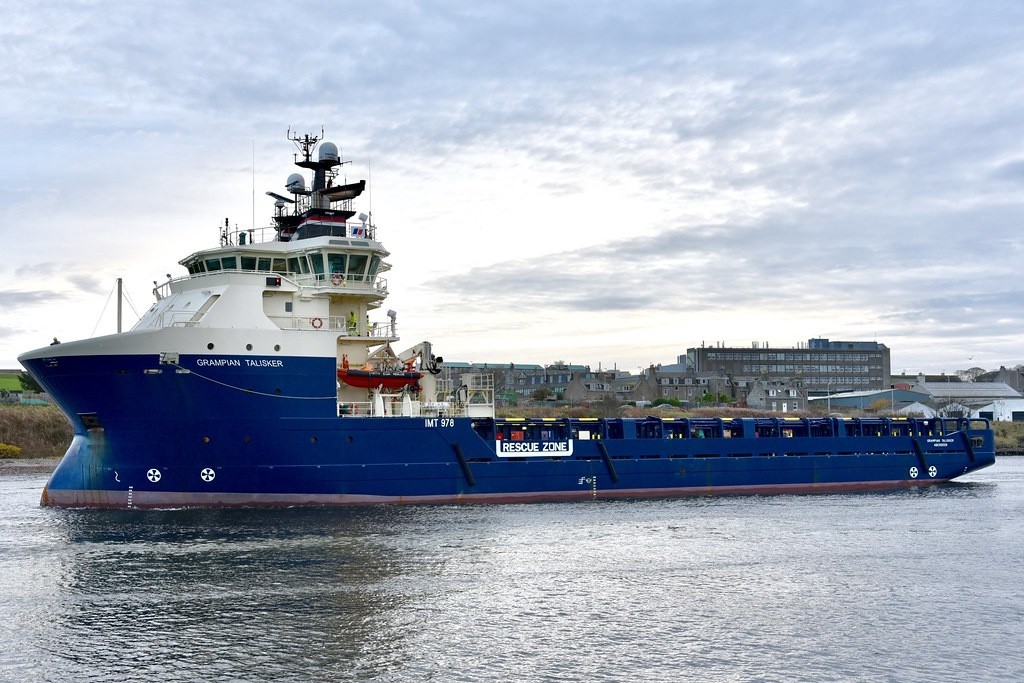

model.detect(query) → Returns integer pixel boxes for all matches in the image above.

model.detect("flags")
[351,226,366,237]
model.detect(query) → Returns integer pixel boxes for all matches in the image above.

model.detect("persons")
[366,315,371,336]
[348,310,360,337]
[574,426,911,458]
[890,381,895,389]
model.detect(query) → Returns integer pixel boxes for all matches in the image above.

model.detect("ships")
[15,123,998,514]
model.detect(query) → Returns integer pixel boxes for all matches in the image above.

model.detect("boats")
[337,367,425,395]
[318,180,366,202]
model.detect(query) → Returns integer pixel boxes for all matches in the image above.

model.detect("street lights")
[827,382,831,418]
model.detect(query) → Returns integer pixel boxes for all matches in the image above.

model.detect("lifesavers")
[331,274,342,285]
[312,318,323,328]
[348,404,359,414]
